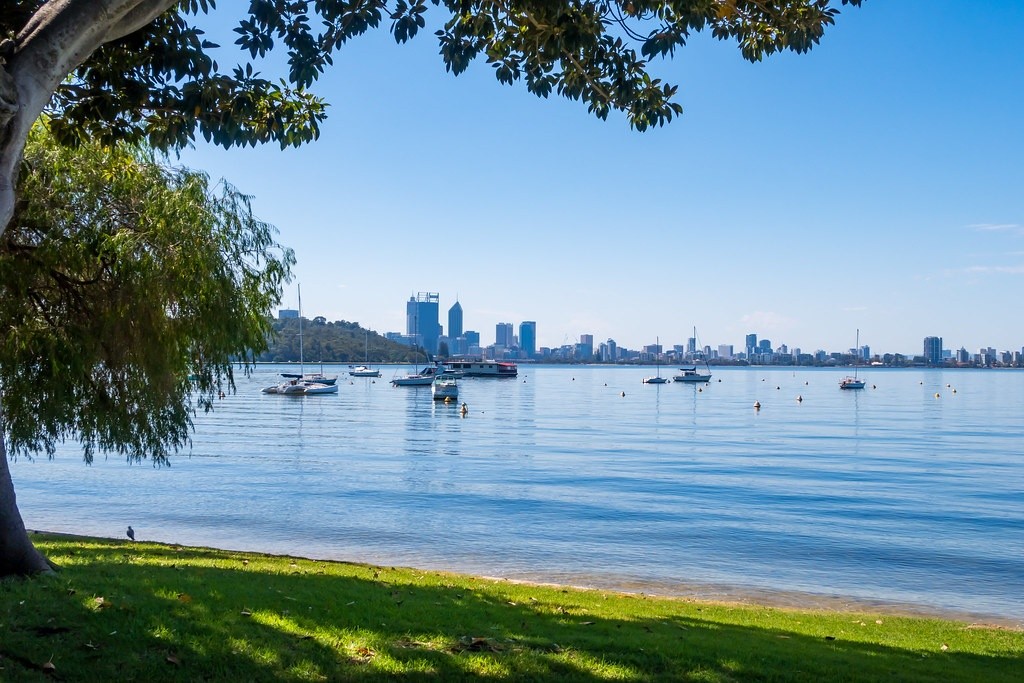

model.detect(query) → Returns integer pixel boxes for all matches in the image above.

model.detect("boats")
[431,375,458,399]
[433,361,519,375]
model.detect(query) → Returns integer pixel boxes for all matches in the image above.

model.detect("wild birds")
[127,526,135,542]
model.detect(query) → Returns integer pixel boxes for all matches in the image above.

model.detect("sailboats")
[673,326,712,381]
[348,332,380,376]
[392,316,436,384]
[261,283,338,393]
[839,328,866,389]
[642,337,668,383]
